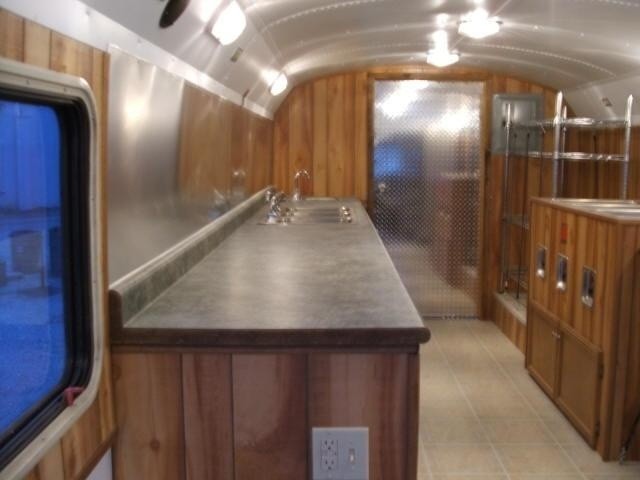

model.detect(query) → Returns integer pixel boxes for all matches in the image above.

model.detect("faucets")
[264,186,288,223]
[292,168,312,201]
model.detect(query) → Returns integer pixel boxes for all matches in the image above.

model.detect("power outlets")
[313,426,344,478]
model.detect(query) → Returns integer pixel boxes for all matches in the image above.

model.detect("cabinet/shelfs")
[501,93,631,306]
[527,197,639,463]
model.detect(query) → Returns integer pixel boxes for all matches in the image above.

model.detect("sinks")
[302,195,337,202]
[289,205,344,215]
[279,215,346,224]
